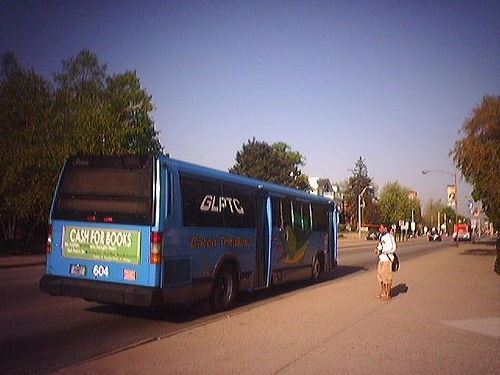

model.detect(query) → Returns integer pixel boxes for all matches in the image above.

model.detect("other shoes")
[376,295,391,300]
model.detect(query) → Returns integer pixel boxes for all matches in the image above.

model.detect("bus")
[40,150,340,316]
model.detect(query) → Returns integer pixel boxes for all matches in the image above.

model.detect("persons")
[372,222,396,300]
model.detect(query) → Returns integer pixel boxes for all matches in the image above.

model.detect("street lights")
[421,169,459,247]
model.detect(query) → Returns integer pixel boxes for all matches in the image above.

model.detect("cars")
[428,233,442,242]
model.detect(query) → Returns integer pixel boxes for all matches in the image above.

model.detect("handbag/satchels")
[392,256,399,271]
